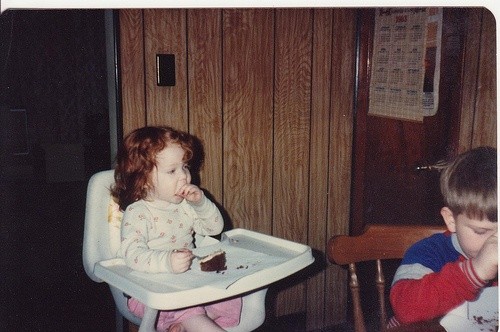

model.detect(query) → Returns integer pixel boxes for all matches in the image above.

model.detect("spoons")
[173,249,209,260]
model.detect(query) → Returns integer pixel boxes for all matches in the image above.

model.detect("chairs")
[81,168,269,332]
[326,224,447,332]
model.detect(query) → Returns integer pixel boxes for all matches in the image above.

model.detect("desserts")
[200,249,227,274]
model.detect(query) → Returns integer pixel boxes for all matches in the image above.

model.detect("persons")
[110,125,244,332]
[383,144,498,332]
